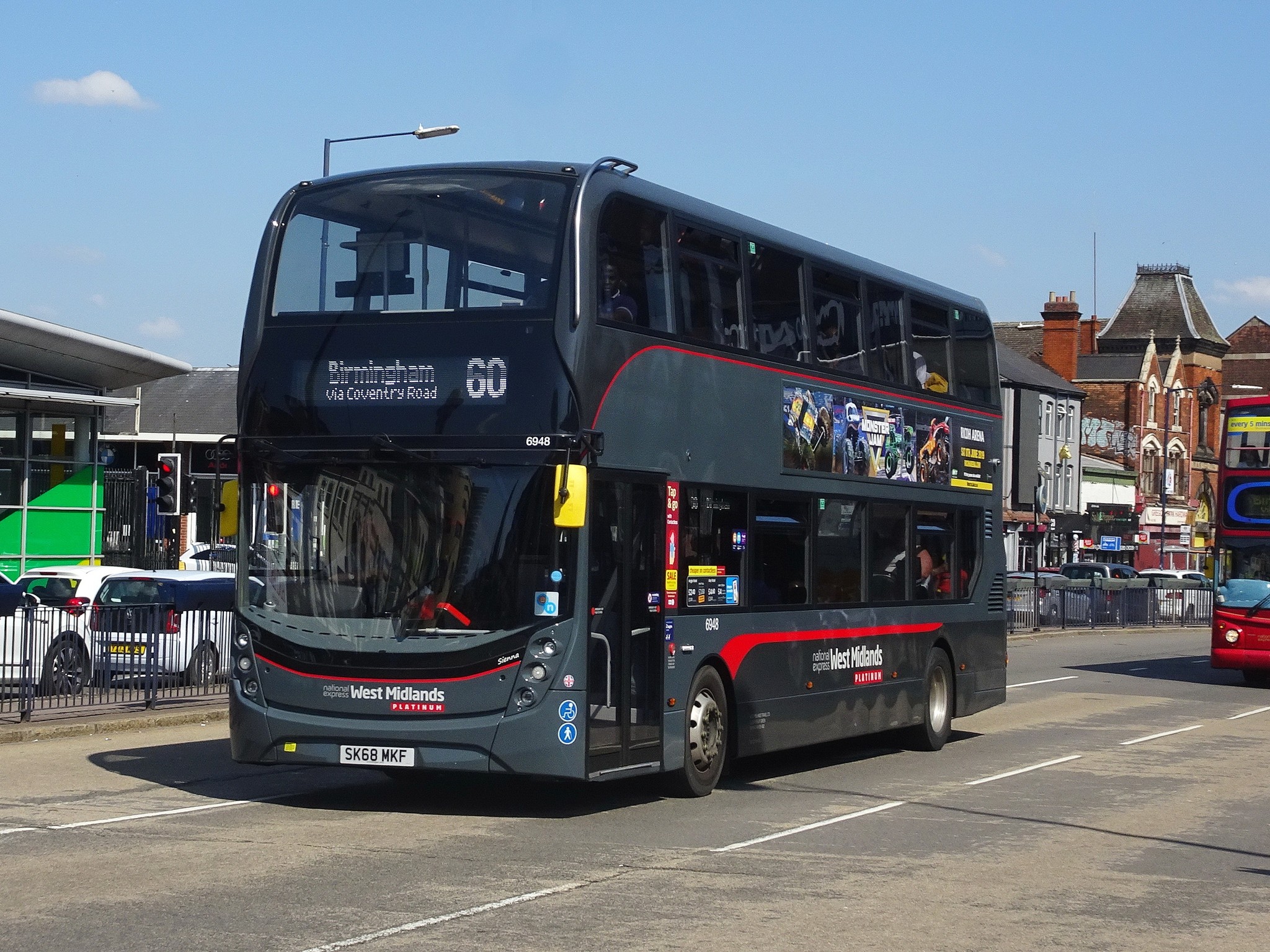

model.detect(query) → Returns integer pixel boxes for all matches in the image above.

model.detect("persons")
[1237,445,1264,467]
[599,262,638,325]
[816,319,864,375]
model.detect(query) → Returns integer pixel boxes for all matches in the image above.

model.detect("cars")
[179,543,314,571]
[0,566,145,697]
[86,570,265,685]
[1006,568,1120,625]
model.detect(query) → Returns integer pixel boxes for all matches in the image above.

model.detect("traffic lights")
[156,453,181,516]
[181,473,198,515]
[264,481,288,536]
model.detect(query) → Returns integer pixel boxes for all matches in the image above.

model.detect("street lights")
[1160,384,1263,572]
[319,123,461,311]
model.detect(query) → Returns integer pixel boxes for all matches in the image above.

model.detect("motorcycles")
[783,387,833,470]
[835,415,871,477]
[920,417,950,482]
[884,405,915,478]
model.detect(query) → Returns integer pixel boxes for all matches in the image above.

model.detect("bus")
[213,157,1006,796]
[1211,397,1270,688]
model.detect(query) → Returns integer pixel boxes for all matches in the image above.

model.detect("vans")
[1058,562,1157,623]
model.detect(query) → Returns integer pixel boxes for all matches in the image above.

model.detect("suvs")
[1135,568,1214,621]
